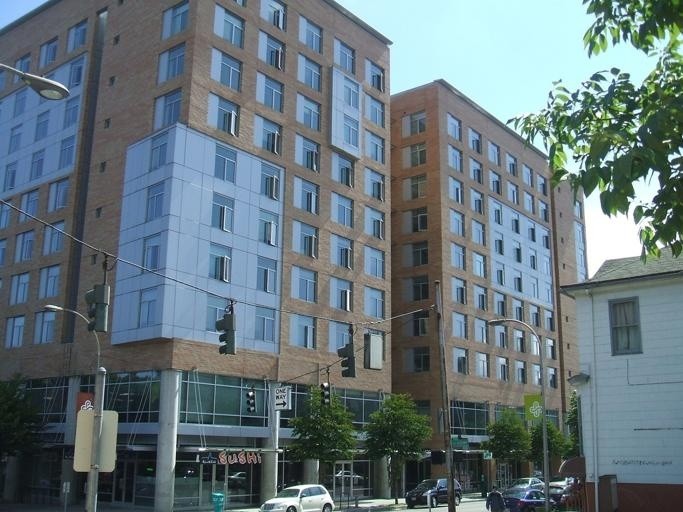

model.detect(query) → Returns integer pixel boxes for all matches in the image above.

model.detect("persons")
[485,486,506,512]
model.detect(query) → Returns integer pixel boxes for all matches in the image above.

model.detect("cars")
[405,478,463,507]
[259,484,335,512]
[500,476,576,512]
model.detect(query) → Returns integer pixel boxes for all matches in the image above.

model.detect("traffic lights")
[214,312,234,355]
[336,344,354,378]
[85,284,111,333]
[246,390,255,413]
[320,381,331,406]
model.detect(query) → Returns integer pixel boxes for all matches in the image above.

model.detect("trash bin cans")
[213,493,224,512]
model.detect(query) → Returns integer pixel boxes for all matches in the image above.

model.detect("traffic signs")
[274,386,291,412]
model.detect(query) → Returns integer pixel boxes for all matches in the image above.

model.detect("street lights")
[45,302,108,512]
[486,318,549,512]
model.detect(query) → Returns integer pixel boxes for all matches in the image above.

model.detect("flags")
[522,393,542,422]
